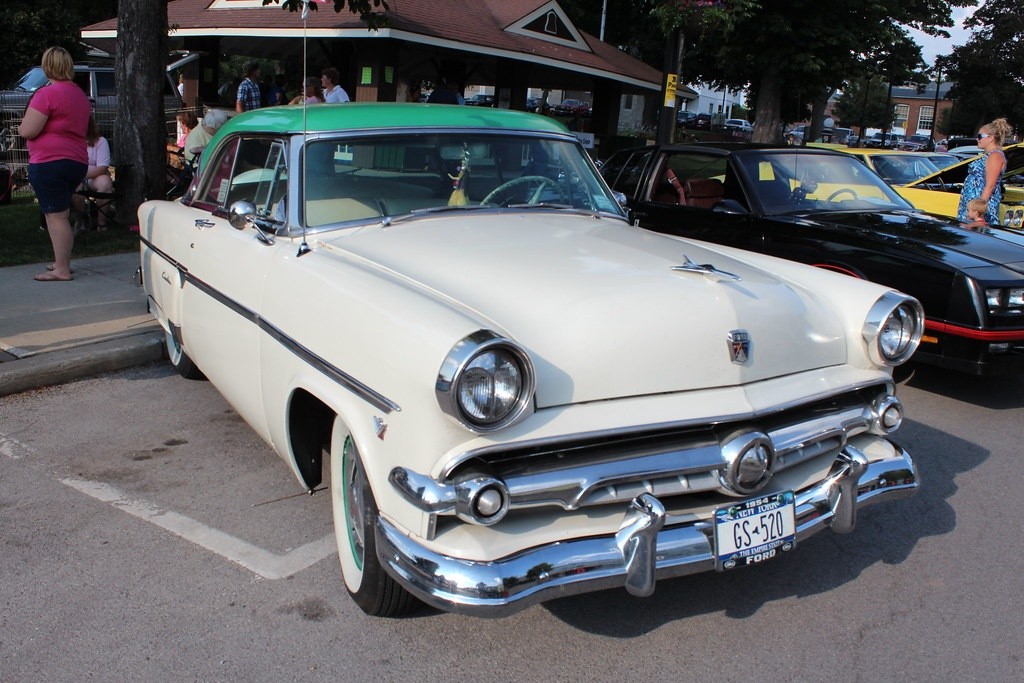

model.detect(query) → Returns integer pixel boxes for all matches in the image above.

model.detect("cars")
[134,101,924,616]
[596,143,1024,375]
[677,109,753,134]
[783,126,1024,181]
[708,143,1024,230]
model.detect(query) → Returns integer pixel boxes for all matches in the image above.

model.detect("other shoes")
[97,224,107,232]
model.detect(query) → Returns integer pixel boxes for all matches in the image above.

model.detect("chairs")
[686,178,725,209]
[757,164,791,207]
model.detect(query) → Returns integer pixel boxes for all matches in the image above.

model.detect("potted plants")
[616,127,652,150]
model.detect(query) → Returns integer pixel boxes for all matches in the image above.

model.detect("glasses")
[976,133,991,139]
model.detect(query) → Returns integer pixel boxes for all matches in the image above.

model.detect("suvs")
[0,49,199,160]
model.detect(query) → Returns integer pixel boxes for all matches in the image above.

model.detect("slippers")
[33,271,73,281]
[46,264,74,274]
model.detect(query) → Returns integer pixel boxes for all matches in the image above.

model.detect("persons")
[18,46,92,281]
[175,60,466,176]
[71,114,113,232]
[957,118,1013,225]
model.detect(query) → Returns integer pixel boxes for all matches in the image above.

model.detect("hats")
[242,57,260,76]
